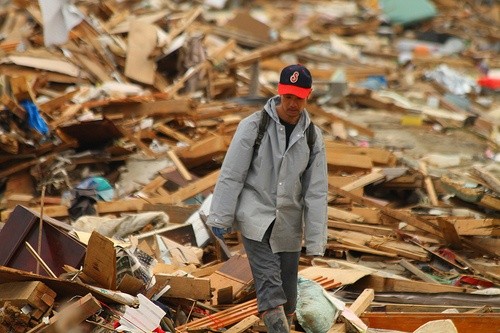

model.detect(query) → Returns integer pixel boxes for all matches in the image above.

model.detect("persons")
[205,64,328,332]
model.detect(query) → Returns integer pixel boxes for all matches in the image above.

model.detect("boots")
[260,305,295,333]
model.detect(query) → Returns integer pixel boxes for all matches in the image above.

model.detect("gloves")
[212,227,232,241]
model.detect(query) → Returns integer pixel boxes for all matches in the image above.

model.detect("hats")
[277,63,313,99]
